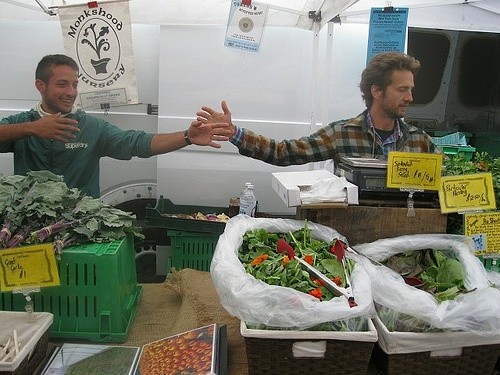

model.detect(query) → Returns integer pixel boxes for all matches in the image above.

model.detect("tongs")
[277,239,354,302]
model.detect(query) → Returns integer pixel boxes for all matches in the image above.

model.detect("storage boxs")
[373,312,500,375]
[164,229,223,276]
[0,310,54,375]
[271,168,359,208]
[435,144,476,162]
[0,231,144,344]
[241,318,379,375]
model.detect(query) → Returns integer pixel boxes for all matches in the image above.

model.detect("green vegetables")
[237,218,367,331]
[441,151,500,212]
[377,249,465,331]
[0,170,145,261]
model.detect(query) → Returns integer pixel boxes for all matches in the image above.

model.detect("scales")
[335,156,438,200]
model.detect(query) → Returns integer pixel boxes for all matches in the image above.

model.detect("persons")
[197,51,451,177]
[0,55,233,199]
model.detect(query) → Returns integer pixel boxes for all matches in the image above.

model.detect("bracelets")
[184,130,192,145]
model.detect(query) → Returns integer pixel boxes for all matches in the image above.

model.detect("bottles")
[239,183,256,218]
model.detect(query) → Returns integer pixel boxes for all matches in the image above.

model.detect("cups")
[229,197,240,217]
